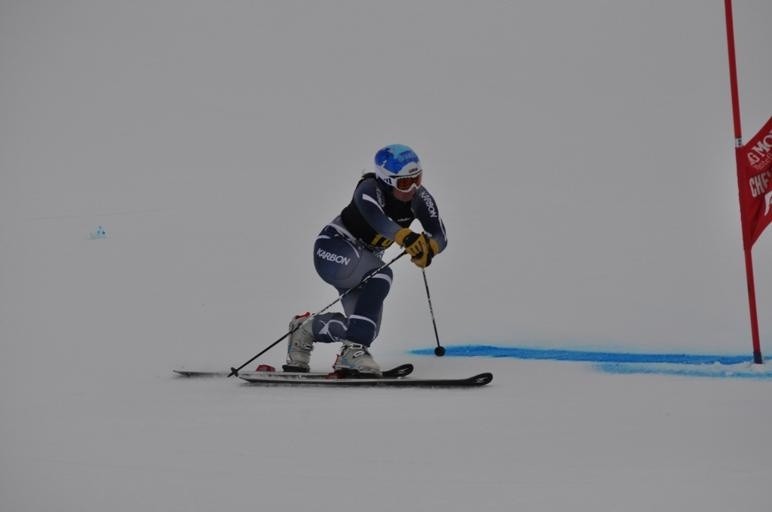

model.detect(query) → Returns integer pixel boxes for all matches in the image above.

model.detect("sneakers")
[286,312,314,365]
[332,344,381,371]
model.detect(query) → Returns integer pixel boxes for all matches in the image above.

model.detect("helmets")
[375,144,423,178]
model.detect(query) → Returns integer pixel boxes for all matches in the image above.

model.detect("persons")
[284,142,447,375]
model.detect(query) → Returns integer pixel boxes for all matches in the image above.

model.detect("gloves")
[411,237,441,268]
[394,228,425,256]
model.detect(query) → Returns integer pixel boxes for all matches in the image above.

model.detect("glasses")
[379,170,423,192]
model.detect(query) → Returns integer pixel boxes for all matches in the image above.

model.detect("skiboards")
[172,363,493,386]
